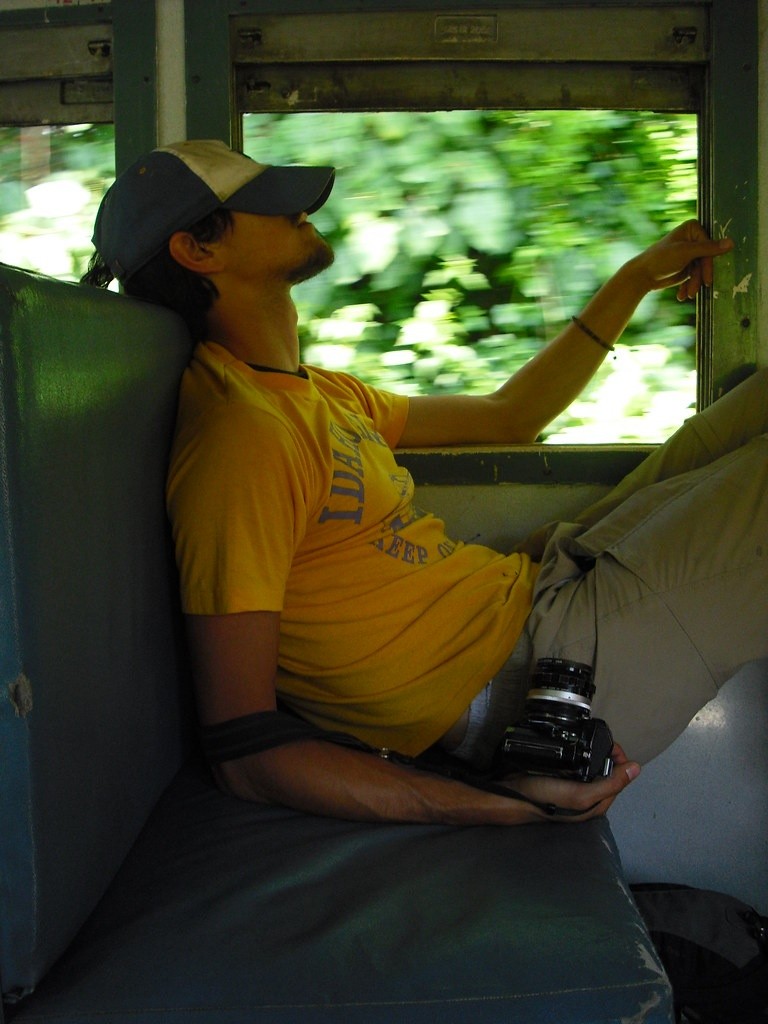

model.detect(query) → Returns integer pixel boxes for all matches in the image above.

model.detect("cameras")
[494,657,613,783]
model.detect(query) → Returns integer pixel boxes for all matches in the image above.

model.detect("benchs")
[0,260,673,1024]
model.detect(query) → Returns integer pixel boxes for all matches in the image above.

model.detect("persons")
[80,140,766,827]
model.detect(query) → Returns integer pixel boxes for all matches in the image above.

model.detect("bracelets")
[573,316,615,351]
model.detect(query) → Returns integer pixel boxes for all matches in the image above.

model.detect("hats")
[91,138,337,285]
